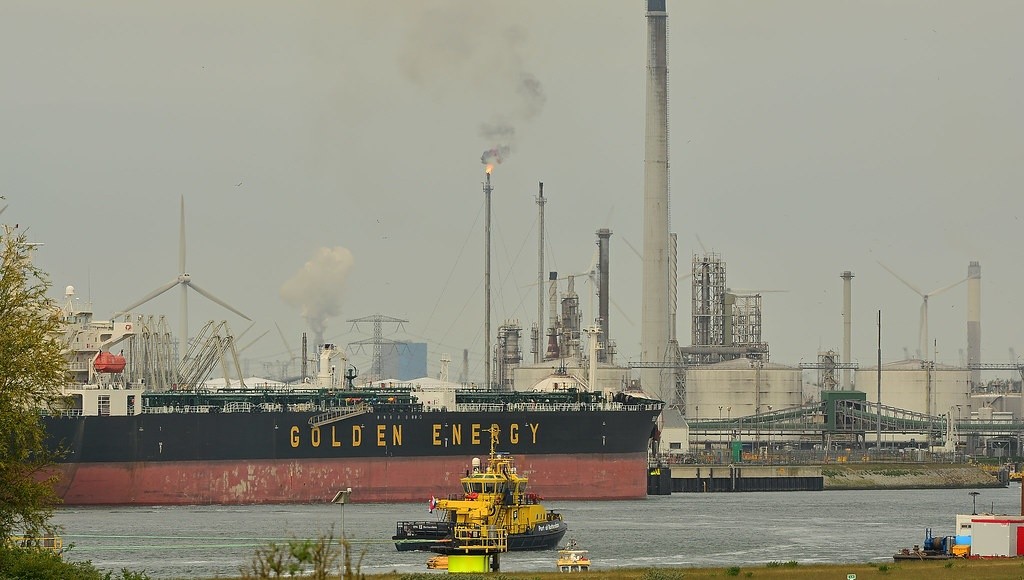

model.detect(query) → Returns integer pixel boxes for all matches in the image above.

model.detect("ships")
[13,387,666,507]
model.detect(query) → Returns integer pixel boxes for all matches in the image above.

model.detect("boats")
[426,523,508,571]
[391,425,567,554]
[892,550,950,561]
[557,536,592,574]
[92,352,126,374]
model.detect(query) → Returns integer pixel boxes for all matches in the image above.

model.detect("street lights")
[718,404,724,451]
[767,404,772,451]
[696,405,700,453]
[958,406,961,445]
[727,406,731,450]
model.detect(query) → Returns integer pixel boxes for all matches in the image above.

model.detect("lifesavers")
[527,493,541,500]
